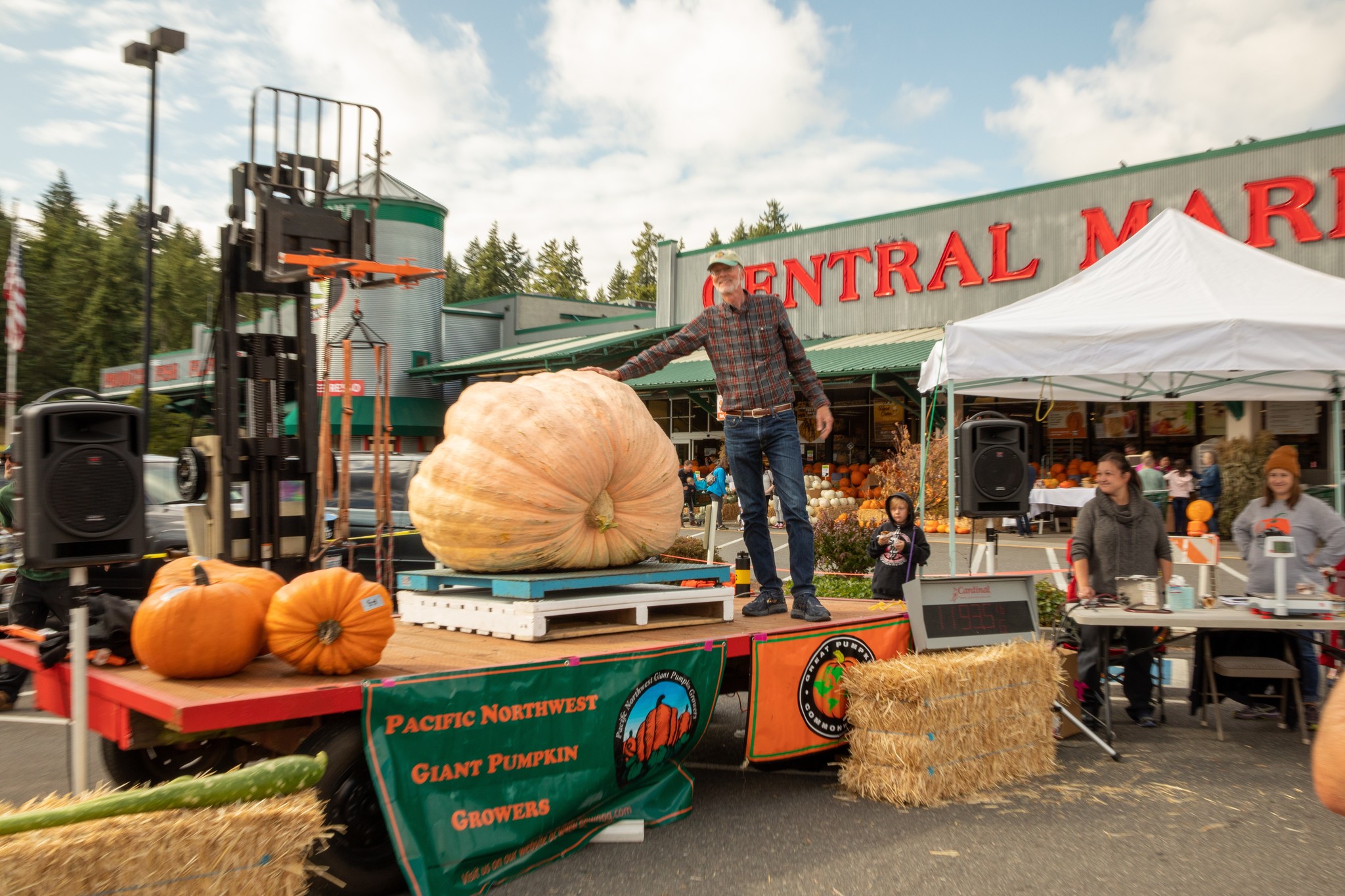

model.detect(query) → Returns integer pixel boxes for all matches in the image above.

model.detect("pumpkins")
[133,556,395,681]
[0,750,328,836]
[679,458,1127,534]
[407,367,684,574]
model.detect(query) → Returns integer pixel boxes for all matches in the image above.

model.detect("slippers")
[737,528,744,531]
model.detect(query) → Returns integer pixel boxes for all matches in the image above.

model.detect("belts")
[725,402,793,418]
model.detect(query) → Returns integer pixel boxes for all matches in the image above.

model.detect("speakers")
[954,409,1034,520]
[8,386,152,573]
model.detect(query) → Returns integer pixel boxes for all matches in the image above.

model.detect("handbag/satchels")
[769,493,773,500]
[706,473,716,486]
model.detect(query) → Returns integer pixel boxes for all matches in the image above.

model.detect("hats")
[707,249,744,271]
[1263,446,1302,479]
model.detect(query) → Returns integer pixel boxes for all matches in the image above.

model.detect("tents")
[915,206,1345,578]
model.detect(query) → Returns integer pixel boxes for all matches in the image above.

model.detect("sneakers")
[1130,715,1160,727]
[1303,702,1319,730]
[1234,704,1281,720]
[690,511,695,518]
[790,593,831,621]
[778,523,784,529]
[1082,716,1097,730]
[772,522,779,528]
[689,522,699,527]
[742,591,788,616]
[715,524,718,530]
[717,524,729,531]
[680,522,685,528]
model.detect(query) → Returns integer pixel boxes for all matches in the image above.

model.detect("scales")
[1243,536,1334,619]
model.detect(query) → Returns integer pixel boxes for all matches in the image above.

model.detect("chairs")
[1198,629,1311,745]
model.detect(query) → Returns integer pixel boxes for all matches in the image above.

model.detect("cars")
[326,454,433,539]
[143,452,246,572]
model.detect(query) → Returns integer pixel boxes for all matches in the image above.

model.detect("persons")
[765,479,784,529]
[762,462,775,514]
[682,477,696,517]
[867,492,931,601]
[0,444,71,711]
[1124,443,1139,455]
[706,458,729,530]
[1230,444,1345,729]
[677,460,699,528]
[576,248,834,621]
[1133,450,1223,539]
[705,453,718,473]
[1069,452,1173,730]
[730,488,745,531]
[1015,453,1036,538]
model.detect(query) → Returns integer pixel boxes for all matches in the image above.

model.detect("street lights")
[121,27,183,454]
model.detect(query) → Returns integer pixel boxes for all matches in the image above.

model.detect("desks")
[1063,600,1345,748]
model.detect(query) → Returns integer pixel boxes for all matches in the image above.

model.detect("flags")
[1,232,27,351]
